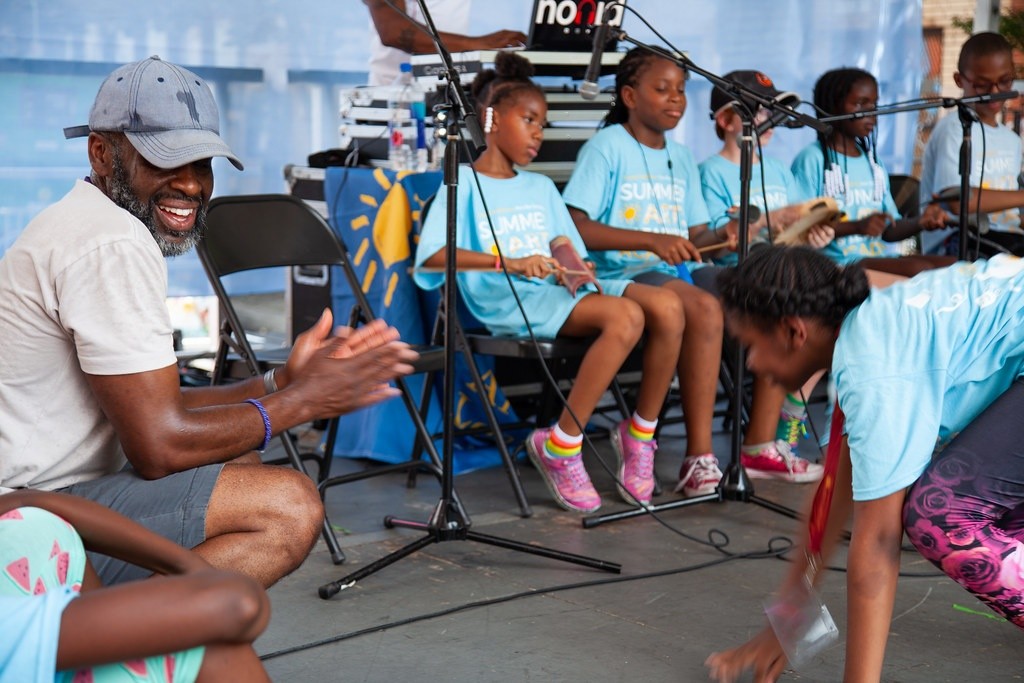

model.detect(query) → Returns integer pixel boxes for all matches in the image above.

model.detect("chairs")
[198,194,472,562]
[404,190,663,516]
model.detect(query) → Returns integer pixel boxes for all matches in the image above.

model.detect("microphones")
[736,102,803,148]
[581,6,620,101]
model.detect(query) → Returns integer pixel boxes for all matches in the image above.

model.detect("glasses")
[960,73,1017,96]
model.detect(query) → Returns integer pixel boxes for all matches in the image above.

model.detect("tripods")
[584,29,855,539]
[320,1,622,599]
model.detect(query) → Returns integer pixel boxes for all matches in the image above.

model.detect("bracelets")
[496,255,501,269]
[243,398,271,454]
[264,368,279,394]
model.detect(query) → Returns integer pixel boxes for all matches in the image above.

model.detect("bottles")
[387,63,453,171]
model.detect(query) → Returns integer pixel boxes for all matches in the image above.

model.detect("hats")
[64,55,244,171]
[710,71,800,120]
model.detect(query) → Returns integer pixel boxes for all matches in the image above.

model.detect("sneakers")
[611,418,658,506]
[676,453,723,498]
[740,439,824,484]
[526,426,602,513]
[776,408,808,458]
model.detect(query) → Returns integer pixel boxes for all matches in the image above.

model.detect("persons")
[414,53,686,514]
[0,489,272,683]
[366,0,529,87]
[919,32,1024,263]
[563,45,826,498]
[790,66,957,278]
[704,240,1024,683]
[695,70,844,459]
[0,55,421,589]
